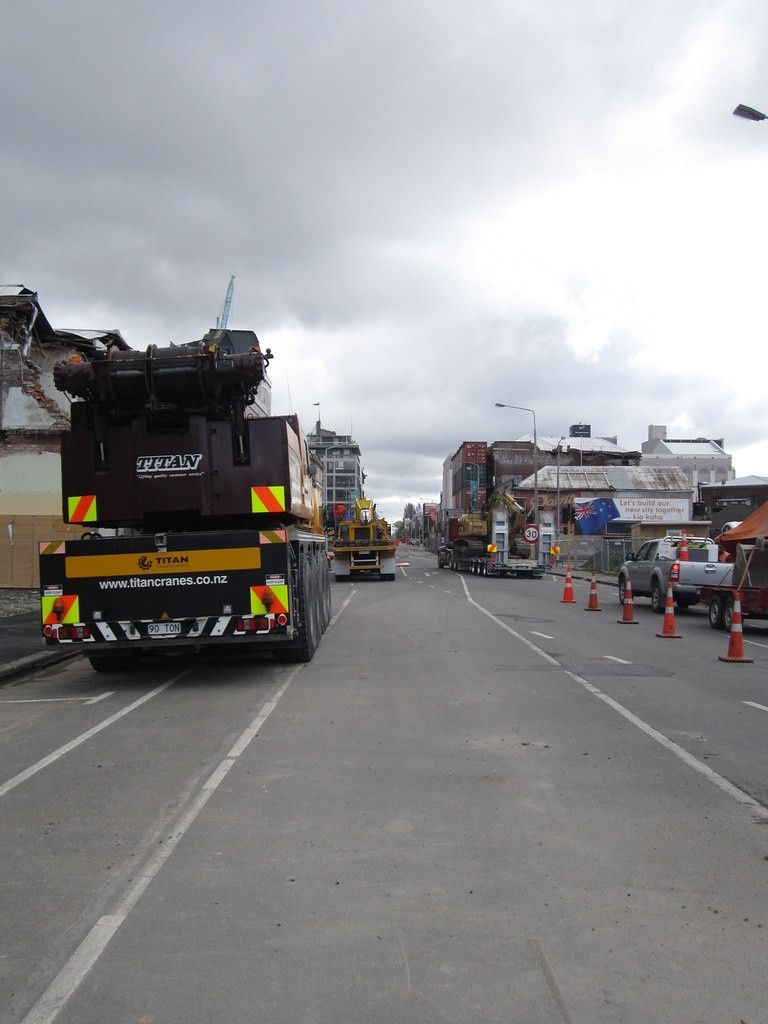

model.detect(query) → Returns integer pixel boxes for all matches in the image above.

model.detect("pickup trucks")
[617,534,736,613]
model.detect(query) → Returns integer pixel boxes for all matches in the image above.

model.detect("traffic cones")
[655,581,683,639]
[617,576,640,624]
[560,565,577,604]
[719,591,754,663]
[679,527,689,561]
[583,571,602,612]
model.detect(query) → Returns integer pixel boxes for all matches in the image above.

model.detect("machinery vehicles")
[331,498,399,582]
[38,328,335,673]
[452,489,531,560]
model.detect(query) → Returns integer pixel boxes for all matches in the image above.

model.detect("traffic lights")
[570,507,576,524]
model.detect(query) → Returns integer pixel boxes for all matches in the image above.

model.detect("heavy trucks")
[437,515,548,579]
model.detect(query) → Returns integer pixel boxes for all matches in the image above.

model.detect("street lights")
[555,435,567,562]
[458,460,480,508]
[494,402,539,560]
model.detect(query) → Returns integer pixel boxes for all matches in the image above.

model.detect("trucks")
[699,543,768,632]
